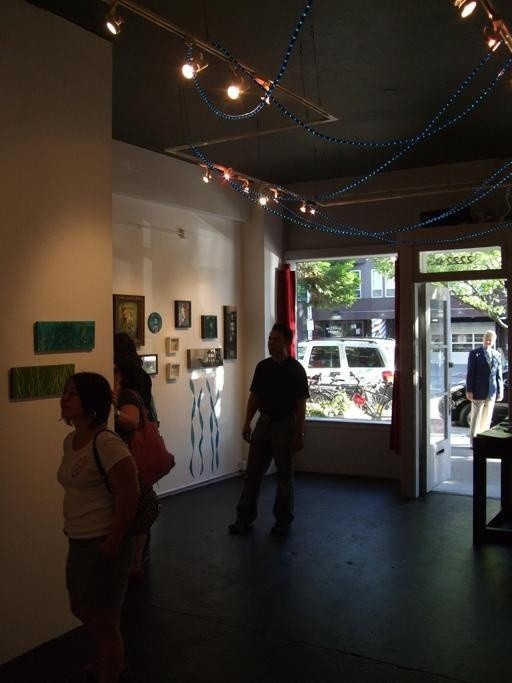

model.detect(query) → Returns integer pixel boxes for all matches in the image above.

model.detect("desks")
[473,416,512,550]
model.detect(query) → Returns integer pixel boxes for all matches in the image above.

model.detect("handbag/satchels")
[132,483,163,536]
[124,419,176,485]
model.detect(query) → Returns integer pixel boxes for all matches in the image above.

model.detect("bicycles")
[305,370,394,420]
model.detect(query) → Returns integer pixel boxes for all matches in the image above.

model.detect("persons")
[228,322,311,537]
[56,371,141,683]
[151,316,159,332]
[465,330,504,450]
[179,303,189,326]
[111,331,161,581]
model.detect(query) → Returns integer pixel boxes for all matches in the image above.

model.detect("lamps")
[453,0,502,52]
[106,0,242,101]
[201,166,317,215]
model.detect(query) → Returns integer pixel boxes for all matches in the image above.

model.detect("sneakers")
[270,528,289,539]
[229,523,254,536]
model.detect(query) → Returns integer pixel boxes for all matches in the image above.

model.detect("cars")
[438,364,508,426]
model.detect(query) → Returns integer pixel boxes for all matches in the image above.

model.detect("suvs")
[296,337,396,401]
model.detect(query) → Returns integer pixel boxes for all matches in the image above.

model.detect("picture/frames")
[141,354,158,375]
[174,301,191,328]
[148,312,162,333]
[201,315,217,338]
[112,294,144,346]
[224,305,237,359]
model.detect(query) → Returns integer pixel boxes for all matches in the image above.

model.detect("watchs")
[296,432,304,438]
[115,409,120,418]
